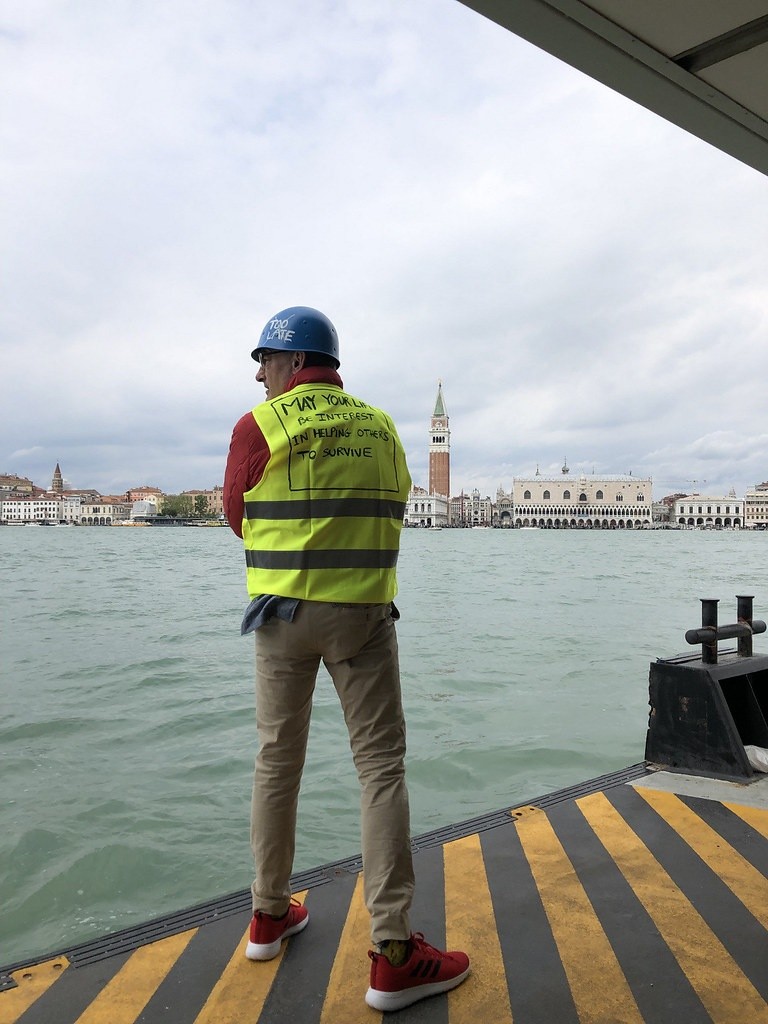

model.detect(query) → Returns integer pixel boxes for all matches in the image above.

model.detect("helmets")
[250,306,340,370]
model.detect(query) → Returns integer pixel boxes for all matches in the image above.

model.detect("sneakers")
[365,930,470,1012]
[245,897,309,960]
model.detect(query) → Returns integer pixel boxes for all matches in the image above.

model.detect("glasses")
[257,349,288,366]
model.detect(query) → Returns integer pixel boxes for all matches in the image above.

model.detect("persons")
[222,308,469,1009]
